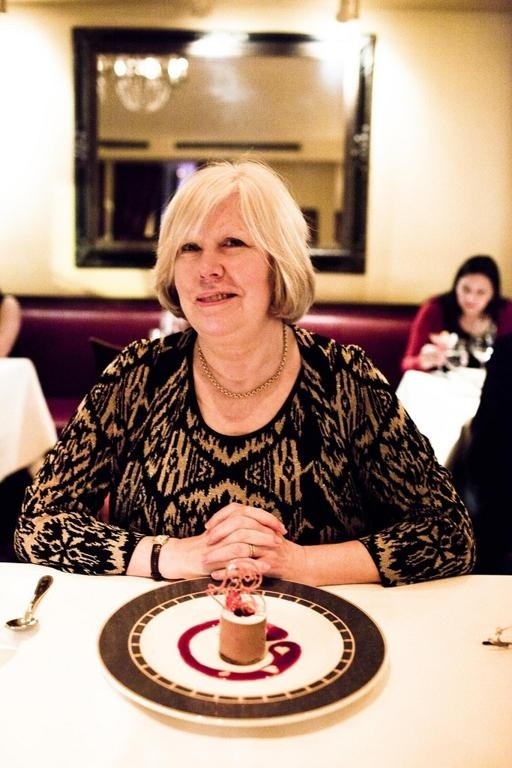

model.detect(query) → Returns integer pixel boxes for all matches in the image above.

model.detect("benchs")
[6,294,420,437]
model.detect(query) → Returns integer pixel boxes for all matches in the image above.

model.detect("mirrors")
[71,26,375,273]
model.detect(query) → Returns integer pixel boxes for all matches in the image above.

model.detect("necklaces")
[195,322,291,400]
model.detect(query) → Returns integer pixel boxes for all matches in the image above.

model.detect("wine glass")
[469,322,497,368]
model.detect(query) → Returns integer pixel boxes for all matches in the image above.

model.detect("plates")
[94,572,388,727]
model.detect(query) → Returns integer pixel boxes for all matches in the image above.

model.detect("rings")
[249,543,255,557]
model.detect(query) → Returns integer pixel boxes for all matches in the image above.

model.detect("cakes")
[205,558,268,666]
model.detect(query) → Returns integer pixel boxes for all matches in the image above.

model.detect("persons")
[13,159,477,587]
[400,255,501,517]
[0,292,23,359]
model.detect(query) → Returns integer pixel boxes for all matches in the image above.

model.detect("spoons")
[6,570,56,634]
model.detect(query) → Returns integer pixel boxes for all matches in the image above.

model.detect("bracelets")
[151,533,170,585]
